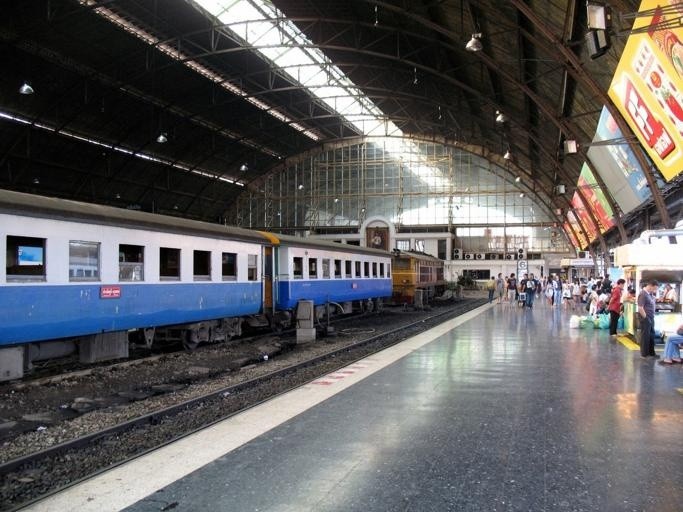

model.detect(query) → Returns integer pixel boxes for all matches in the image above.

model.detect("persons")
[605,277,624,336]
[485,271,613,318]
[637,279,661,360]
[658,323,683,365]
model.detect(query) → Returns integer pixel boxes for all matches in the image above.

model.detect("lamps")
[466,0,525,199]
[27,177,366,214]
[19,80,34,94]
[157,120,167,142]
[240,164,248,171]
[552,0,683,236]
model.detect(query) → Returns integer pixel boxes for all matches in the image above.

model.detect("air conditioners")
[454,248,527,260]
[577,251,590,259]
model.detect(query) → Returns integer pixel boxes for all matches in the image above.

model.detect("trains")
[0,189,448,383]
